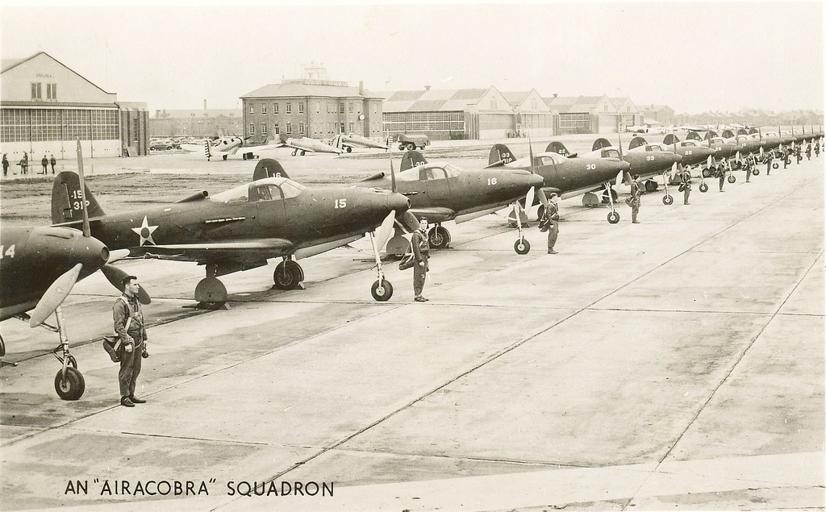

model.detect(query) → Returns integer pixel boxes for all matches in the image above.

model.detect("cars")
[150,136,202,150]
[630,122,746,132]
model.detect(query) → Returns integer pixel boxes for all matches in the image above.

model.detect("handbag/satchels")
[714,170,721,178]
[538,215,550,233]
[678,184,685,192]
[103,336,125,362]
[400,254,415,270]
[625,197,633,207]
[741,164,749,171]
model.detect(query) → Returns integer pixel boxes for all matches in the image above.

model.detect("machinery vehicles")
[396,133,431,150]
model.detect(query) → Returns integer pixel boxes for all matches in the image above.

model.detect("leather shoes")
[548,250,556,254]
[121,396,134,407]
[415,297,425,302]
[131,394,146,403]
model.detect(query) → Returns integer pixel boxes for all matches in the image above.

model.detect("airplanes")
[47,147,420,310]
[281,133,344,159]
[326,130,389,152]
[201,133,253,160]
[350,151,549,256]
[488,136,634,224]
[1,138,154,400]
[546,125,826,204]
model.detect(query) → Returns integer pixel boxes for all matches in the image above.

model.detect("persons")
[112,275,146,407]
[682,164,691,205]
[50,153,56,174]
[2,153,9,176]
[630,175,640,224]
[412,216,430,303]
[546,192,559,254]
[41,155,48,175]
[766,140,824,175]
[20,156,26,175]
[718,156,727,192]
[746,152,753,183]
